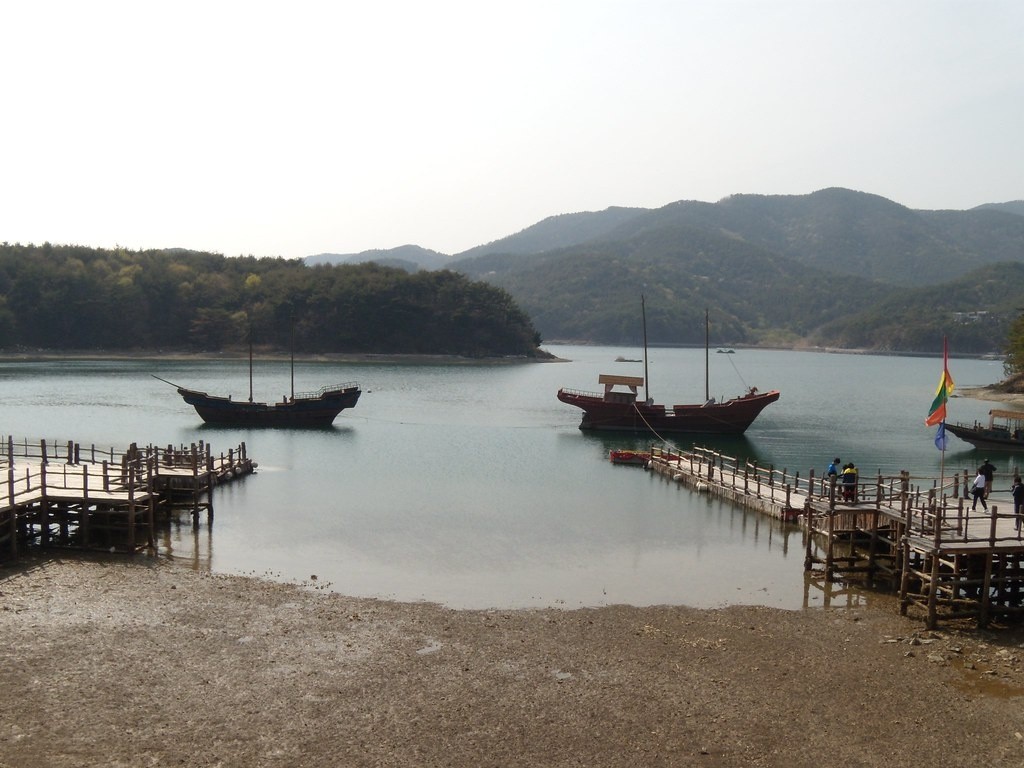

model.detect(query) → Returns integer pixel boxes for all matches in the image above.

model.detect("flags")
[926,352,955,426]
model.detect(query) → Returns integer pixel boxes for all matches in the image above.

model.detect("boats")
[935,409,1024,452]
[149,322,362,428]
[556,295,780,436]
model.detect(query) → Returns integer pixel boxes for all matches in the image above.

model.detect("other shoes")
[969,509,976,512]
[1014,528,1018,530]
[983,509,989,512]
[984,496,987,499]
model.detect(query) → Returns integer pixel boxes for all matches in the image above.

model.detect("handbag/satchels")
[970,485,977,495]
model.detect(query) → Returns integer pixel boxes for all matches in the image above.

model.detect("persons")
[828,458,858,502]
[968,470,989,513]
[1010,476,1024,530]
[978,458,997,493]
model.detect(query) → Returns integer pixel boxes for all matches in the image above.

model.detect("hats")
[984,459,989,463]
